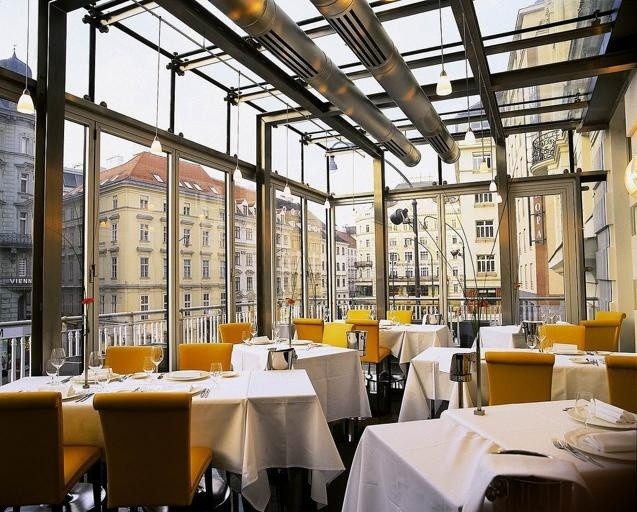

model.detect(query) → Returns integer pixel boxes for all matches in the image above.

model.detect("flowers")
[47,193,94,332]
[287,253,297,306]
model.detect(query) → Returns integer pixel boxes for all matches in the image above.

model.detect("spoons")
[156,374,163,379]
[553,439,588,463]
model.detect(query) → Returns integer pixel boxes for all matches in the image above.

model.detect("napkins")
[584,431,637,453]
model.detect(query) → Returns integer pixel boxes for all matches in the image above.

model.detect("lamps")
[435,0,503,204]
[351,148,357,216]
[18,0,35,115]
[233,70,244,182]
[150,17,163,156]
[323,128,331,209]
[283,102,292,199]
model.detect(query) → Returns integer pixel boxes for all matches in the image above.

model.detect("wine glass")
[526,334,536,350]
[210,362,223,394]
[574,392,596,429]
[536,325,546,352]
[272,329,280,348]
[45,347,65,388]
[143,356,154,384]
[151,344,163,379]
[88,352,110,392]
[271,321,280,350]
[249,323,257,349]
[240,331,249,348]
[538,307,555,325]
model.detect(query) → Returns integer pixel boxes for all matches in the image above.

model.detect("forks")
[562,442,603,468]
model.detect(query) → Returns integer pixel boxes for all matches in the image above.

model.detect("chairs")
[0,391,100,511]
[176,343,236,372]
[104,346,163,370]
[93,392,214,512]
[218,310,636,430]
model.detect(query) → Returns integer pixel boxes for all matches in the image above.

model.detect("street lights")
[423,213,467,321]
[389,198,421,307]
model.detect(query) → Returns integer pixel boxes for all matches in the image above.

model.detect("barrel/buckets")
[523,321,544,343]
[346,330,368,356]
[267,348,297,370]
[427,314,443,325]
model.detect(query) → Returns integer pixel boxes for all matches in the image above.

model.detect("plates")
[61,389,82,400]
[164,370,210,380]
[285,339,312,345]
[562,428,636,462]
[212,371,236,377]
[561,406,636,428]
[552,350,585,355]
[568,356,598,364]
[129,373,146,378]
[249,339,275,345]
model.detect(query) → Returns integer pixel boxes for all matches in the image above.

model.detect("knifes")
[107,373,132,383]
[81,392,94,402]
[200,386,210,398]
